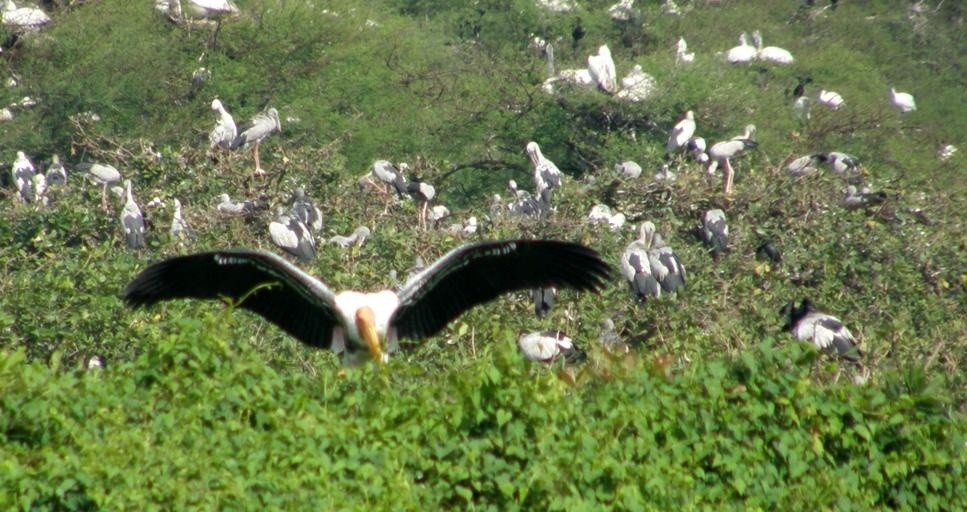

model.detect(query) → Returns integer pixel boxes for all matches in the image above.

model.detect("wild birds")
[1,0,51,28]
[10,149,195,258]
[778,297,866,389]
[673,38,695,67]
[230,107,282,180]
[156,0,242,51]
[817,87,845,111]
[117,238,621,372]
[792,84,813,121]
[726,30,795,65]
[215,141,562,266]
[890,87,918,112]
[532,287,558,322]
[778,149,887,212]
[586,110,756,301]
[517,327,588,369]
[207,98,238,162]
[541,43,657,105]
[188,52,212,100]
